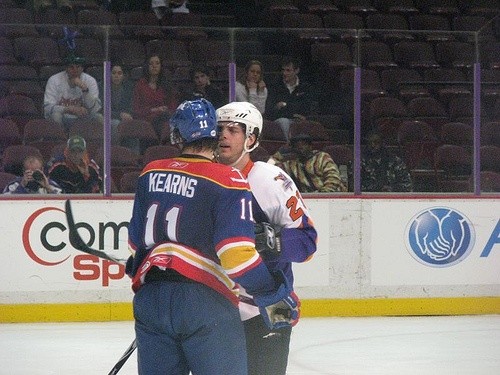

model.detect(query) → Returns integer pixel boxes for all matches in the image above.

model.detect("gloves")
[255,221,281,254]
[254,278,301,330]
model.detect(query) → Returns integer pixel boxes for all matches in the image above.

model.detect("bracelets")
[82,88,89,92]
[283,102,287,107]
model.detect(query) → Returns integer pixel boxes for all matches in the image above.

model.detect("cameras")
[32,170,42,181]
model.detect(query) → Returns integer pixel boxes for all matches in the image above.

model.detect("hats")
[67,135,87,151]
[288,134,312,147]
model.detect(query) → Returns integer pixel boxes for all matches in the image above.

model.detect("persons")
[130,54,178,112]
[110,62,136,143]
[264,57,315,121]
[127,99,301,375]
[347,130,414,192]
[234,60,268,113]
[270,133,346,193]
[181,67,229,110]
[43,52,102,129]
[48,135,103,193]
[214,102,318,375]
[2,156,62,194]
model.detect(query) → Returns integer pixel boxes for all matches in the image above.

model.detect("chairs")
[0,0,500,195]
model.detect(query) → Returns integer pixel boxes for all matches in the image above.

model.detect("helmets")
[214,101,263,139]
[168,97,218,144]
